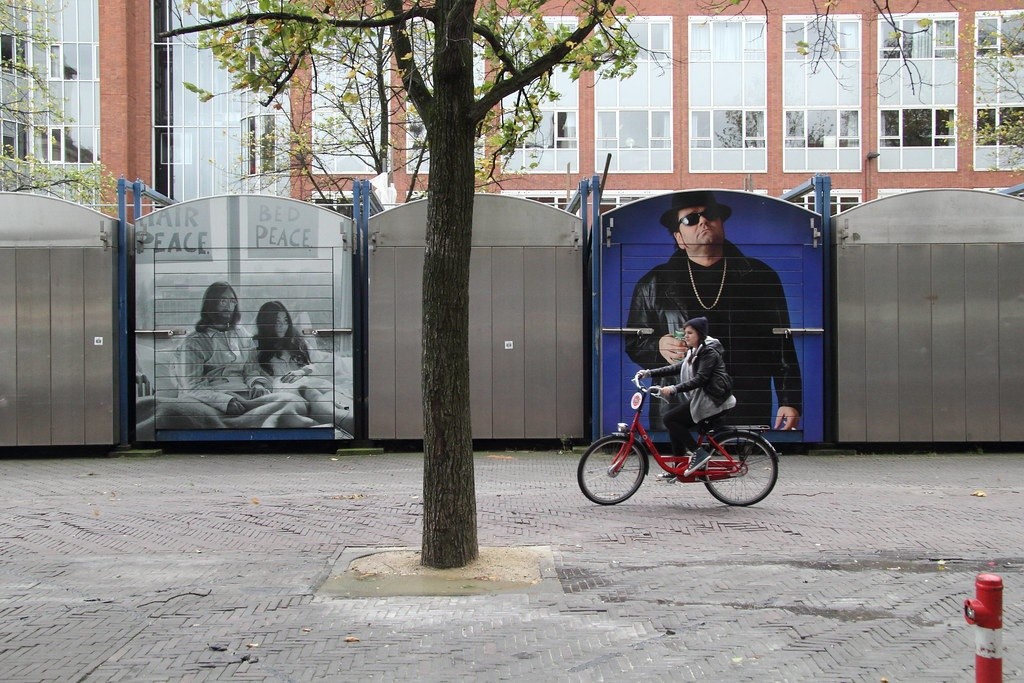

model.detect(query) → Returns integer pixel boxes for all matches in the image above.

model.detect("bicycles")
[575,371,782,508]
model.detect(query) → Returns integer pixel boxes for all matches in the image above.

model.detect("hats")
[660,191,732,229]
[687,317,708,336]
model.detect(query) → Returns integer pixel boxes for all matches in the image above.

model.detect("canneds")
[670,328,688,361]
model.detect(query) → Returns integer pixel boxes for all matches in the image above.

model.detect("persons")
[171,280,267,415]
[622,190,807,442]
[636,316,738,483]
[247,300,355,439]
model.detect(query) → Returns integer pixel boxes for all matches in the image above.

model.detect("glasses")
[672,206,723,232]
[215,299,238,307]
[273,318,289,328]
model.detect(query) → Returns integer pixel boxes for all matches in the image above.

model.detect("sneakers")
[684,447,712,477]
[657,468,676,480]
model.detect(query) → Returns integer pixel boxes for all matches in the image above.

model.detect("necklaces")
[685,256,729,312]
